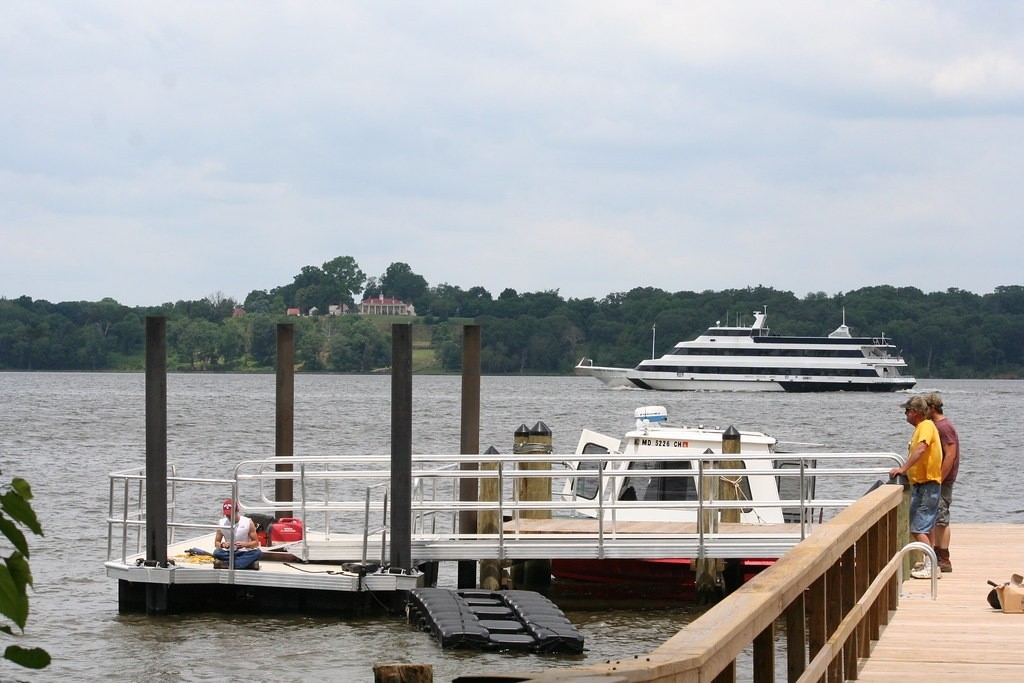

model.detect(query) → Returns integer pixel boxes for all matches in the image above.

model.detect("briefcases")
[270,517,303,541]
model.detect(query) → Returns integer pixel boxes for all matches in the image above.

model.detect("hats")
[923,393,942,406]
[224,499,238,515]
[900,396,927,409]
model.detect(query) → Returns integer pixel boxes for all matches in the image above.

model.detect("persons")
[923,394,959,573]
[889,396,942,579]
[212,500,263,570]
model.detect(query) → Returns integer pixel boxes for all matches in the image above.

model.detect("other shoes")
[214,559,230,568]
[937,561,952,572]
[246,561,260,570]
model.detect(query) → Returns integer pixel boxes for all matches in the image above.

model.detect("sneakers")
[910,565,942,578]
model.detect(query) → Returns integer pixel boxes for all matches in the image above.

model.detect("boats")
[559,403,820,524]
[625,303,917,394]
